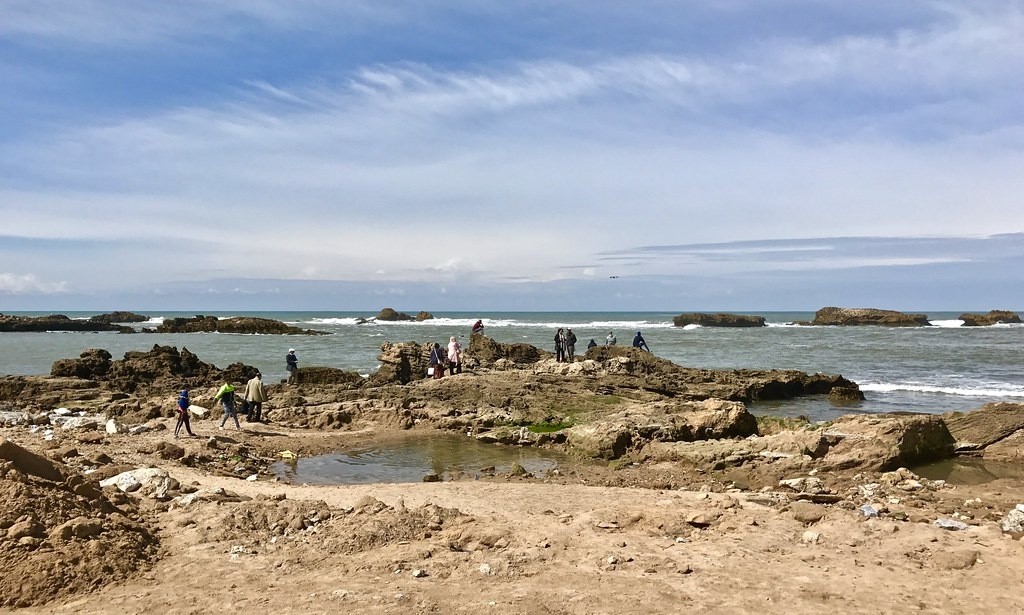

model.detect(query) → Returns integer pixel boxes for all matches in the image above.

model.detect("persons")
[213,377,245,431]
[285,347,298,387]
[473,319,484,335]
[587,338,597,349]
[554,328,567,363]
[430,342,444,380]
[605,331,616,346]
[447,336,463,375]
[565,328,578,363]
[173,384,196,437]
[633,331,651,353]
[244,373,268,422]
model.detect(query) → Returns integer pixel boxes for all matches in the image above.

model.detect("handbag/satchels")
[438,359,443,365]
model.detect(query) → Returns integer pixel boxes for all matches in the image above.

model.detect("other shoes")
[174,433,178,438]
[237,428,245,431]
[189,433,197,436]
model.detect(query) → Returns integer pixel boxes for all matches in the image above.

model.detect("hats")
[566,328,571,331]
[289,348,295,352]
